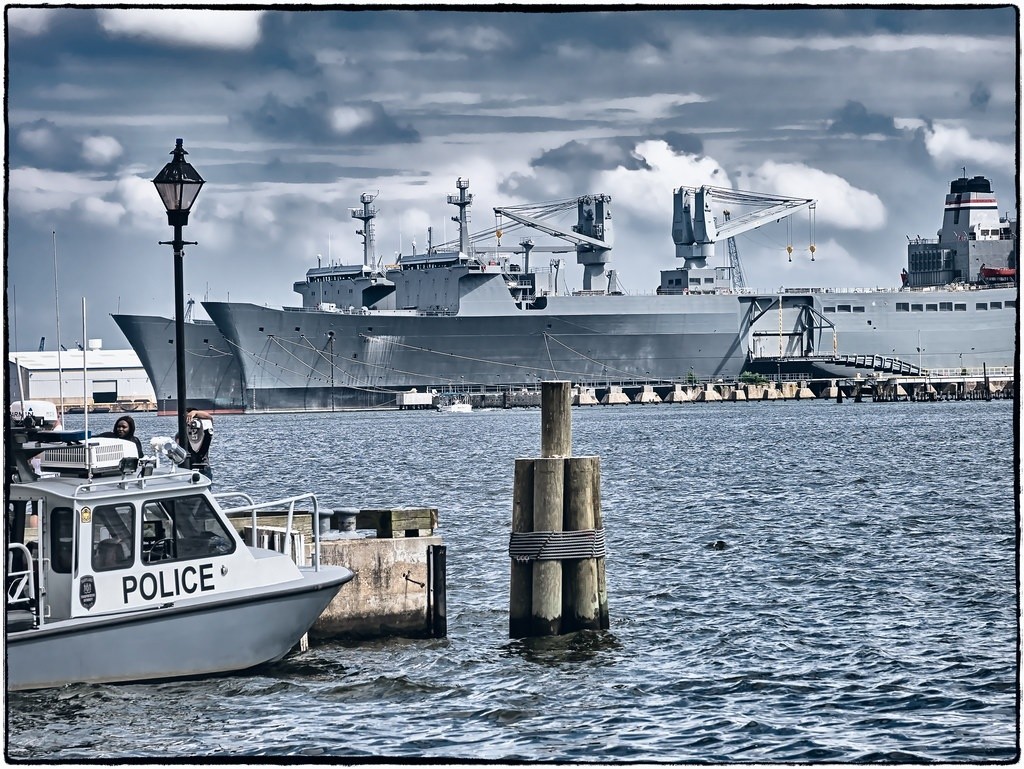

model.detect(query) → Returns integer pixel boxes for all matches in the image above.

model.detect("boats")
[109,174,1017,416]
[8,401,356,691]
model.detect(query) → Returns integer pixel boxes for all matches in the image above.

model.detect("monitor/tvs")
[142,520,162,541]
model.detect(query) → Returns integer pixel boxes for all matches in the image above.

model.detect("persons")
[175,407,213,491]
[113,416,144,458]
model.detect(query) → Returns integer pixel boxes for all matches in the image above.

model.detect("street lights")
[152,139,206,471]
